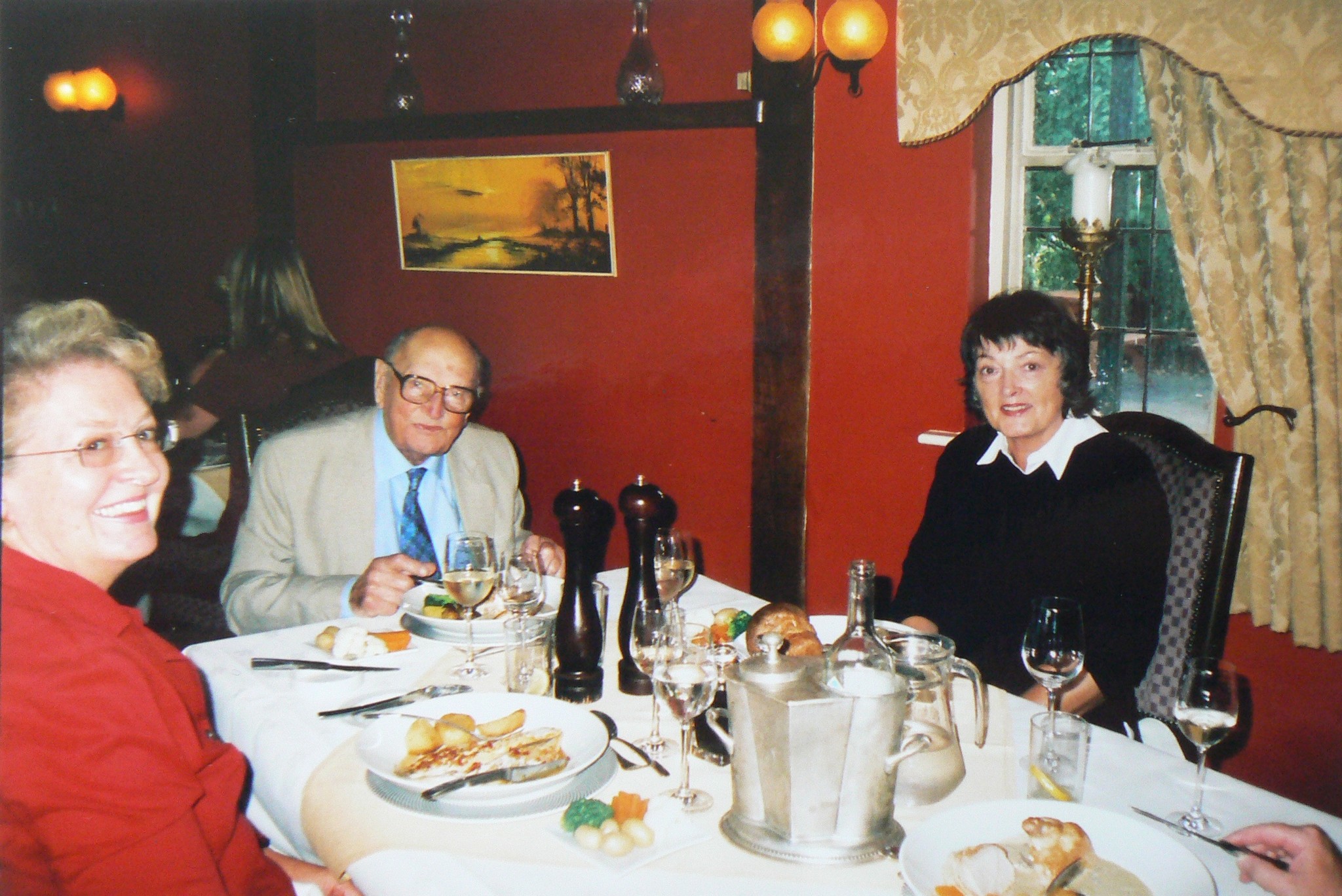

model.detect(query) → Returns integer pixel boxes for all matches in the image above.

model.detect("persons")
[885,290,1172,744]
[152,300,240,536]
[1220,822,1342,896]
[219,325,567,637]
[0,298,362,896]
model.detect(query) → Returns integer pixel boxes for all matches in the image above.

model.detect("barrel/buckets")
[718,659,906,866]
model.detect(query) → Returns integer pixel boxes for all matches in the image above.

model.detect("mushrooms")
[1022,817,1091,856]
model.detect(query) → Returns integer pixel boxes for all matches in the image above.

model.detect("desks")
[175,563,1342,896]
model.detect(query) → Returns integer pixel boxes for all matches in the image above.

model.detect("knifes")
[1130,806,1291,873]
[317,683,473,719]
[251,657,400,672]
[419,759,568,801]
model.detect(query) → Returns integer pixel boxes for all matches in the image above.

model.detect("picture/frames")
[389,149,621,276]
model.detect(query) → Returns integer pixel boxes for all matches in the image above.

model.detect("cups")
[1026,711,1091,803]
[503,617,552,696]
[560,580,607,668]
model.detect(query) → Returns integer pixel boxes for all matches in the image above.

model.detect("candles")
[1063,147,1115,235]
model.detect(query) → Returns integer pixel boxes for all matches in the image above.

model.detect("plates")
[897,799,1218,896]
[730,616,920,678]
[346,691,611,801]
[403,571,564,640]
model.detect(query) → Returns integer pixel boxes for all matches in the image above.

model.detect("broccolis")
[563,798,613,830]
[727,610,752,637]
[332,625,389,661]
[425,595,455,605]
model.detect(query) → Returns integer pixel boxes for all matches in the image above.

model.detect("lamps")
[749,0,891,111]
[37,60,126,134]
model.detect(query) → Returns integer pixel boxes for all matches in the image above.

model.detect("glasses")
[1,420,180,468]
[386,359,481,415]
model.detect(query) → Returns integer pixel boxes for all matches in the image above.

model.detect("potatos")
[316,626,339,648]
[715,608,734,625]
[423,602,464,619]
[405,709,527,753]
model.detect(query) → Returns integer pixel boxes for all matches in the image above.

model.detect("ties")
[399,468,442,586]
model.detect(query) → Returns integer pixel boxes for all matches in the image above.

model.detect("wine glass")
[628,528,720,814]
[1165,657,1240,842]
[1020,596,1086,781]
[442,532,499,679]
[499,547,547,690]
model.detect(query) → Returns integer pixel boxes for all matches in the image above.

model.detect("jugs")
[872,624,991,809]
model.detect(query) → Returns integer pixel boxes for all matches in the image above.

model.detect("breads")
[745,603,824,660]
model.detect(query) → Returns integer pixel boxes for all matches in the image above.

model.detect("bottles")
[808,558,896,698]
[691,645,740,767]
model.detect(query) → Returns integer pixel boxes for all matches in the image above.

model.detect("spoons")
[590,709,671,778]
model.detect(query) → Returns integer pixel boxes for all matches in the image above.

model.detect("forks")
[608,746,652,772]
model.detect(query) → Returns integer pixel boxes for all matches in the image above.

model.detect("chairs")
[871,410,1256,770]
[224,352,383,521]
